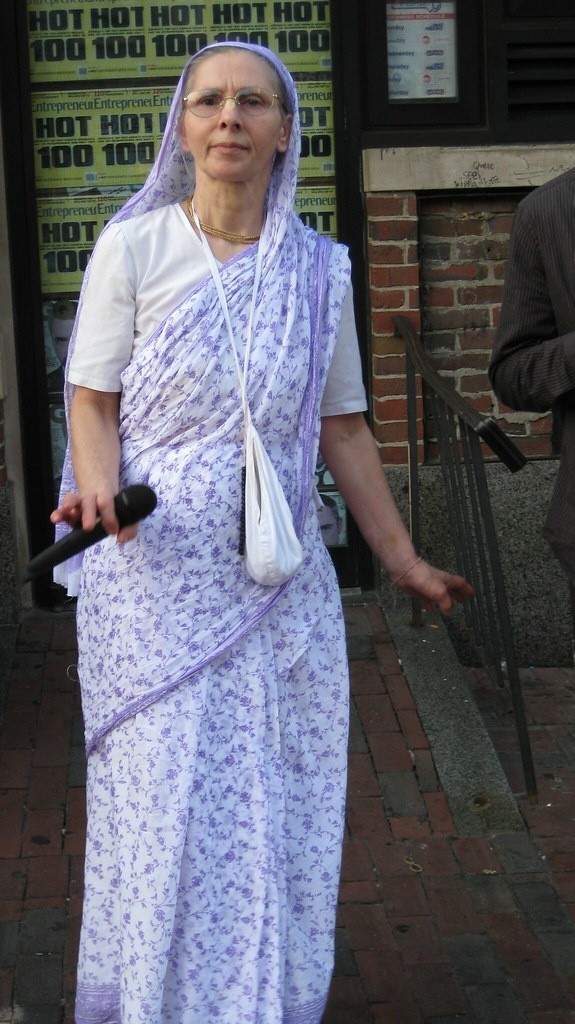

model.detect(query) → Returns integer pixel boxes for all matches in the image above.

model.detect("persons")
[51,43,477,1024]
[488,168,575,690]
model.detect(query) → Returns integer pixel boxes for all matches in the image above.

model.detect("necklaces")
[184,196,261,246]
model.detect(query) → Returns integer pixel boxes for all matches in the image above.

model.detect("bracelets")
[387,555,425,589]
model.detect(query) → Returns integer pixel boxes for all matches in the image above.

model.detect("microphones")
[20,484,157,585]
